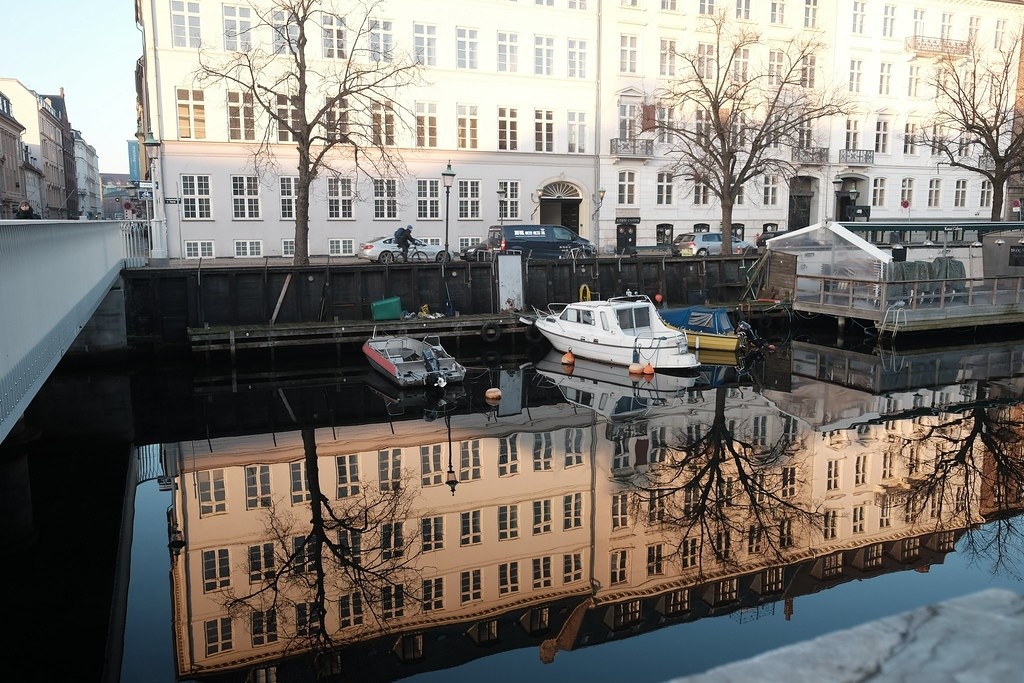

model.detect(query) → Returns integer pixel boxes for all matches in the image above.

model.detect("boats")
[657,305,765,351]
[534,293,702,370]
[362,370,469,422]
[361,326,466,389]
[689,349,759,385]
[531,347,701,422]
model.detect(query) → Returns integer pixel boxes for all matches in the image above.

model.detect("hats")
[407,224,414,230]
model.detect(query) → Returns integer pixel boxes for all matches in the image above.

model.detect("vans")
[485,223,597,260]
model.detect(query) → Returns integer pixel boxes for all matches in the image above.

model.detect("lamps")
[531,189,543,220]
[591,187,607,219]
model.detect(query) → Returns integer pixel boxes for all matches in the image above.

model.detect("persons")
[755,233,760,242]
[394,224,416,262]
[15,200,41,219]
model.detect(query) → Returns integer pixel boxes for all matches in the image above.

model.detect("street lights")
[849,182,859,222]
[442,159,457,263]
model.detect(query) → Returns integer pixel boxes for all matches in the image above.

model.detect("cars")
[670,232,754,258]
[756,231,832,251]
[460,237,501,262]
[357,235,454,264]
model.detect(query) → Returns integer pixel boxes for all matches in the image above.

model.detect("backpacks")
[395,228,408,239]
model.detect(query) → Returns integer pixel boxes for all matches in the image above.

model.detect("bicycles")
[388,242,429,264]
